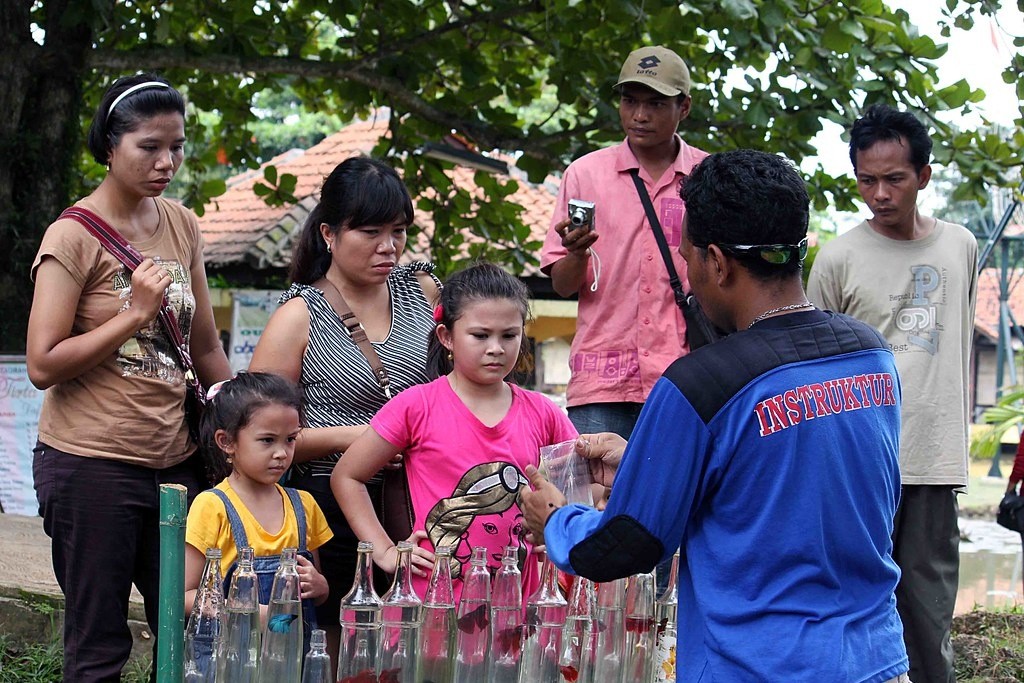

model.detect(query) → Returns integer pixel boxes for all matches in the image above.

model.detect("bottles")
[522,549,565,683]
[596,580,626,683]
[302,629,333,683]
[422,546,454,683]
[260,548,304,683]
[654,548,679,683]
[494,547,521,683]
[216,547,257,683]
[456,547,490,683]
[380,541,423,683]
[183,547,224,683]
[560,576,598,683]
[337,541,383,683]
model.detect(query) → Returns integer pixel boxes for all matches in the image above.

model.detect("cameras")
[569,199,596,236]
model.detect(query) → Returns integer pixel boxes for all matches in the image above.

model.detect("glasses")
[713,235,809,266]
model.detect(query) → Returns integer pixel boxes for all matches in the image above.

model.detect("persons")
[518,146,917,683]
[329,263,607,666]
[26,73,238,683]
[806,103,981,682]
[183,368,335,661]
[248,157,450,683]
[541,45,728,682]
[1006,429,1024,600]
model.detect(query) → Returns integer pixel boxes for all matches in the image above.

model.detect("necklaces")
[747,301,813,329]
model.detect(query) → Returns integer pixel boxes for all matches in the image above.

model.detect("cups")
[541,445,595,511]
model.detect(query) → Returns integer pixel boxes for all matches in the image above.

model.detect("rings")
[156,273,164,281]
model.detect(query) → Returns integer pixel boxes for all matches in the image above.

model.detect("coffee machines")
[626,573,654,683]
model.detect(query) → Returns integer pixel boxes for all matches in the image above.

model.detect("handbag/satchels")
[183,379,227,487]
[678,292,728,352]
[380,461,416,546]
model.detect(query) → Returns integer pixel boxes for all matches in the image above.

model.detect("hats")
[612,46,691,96]
